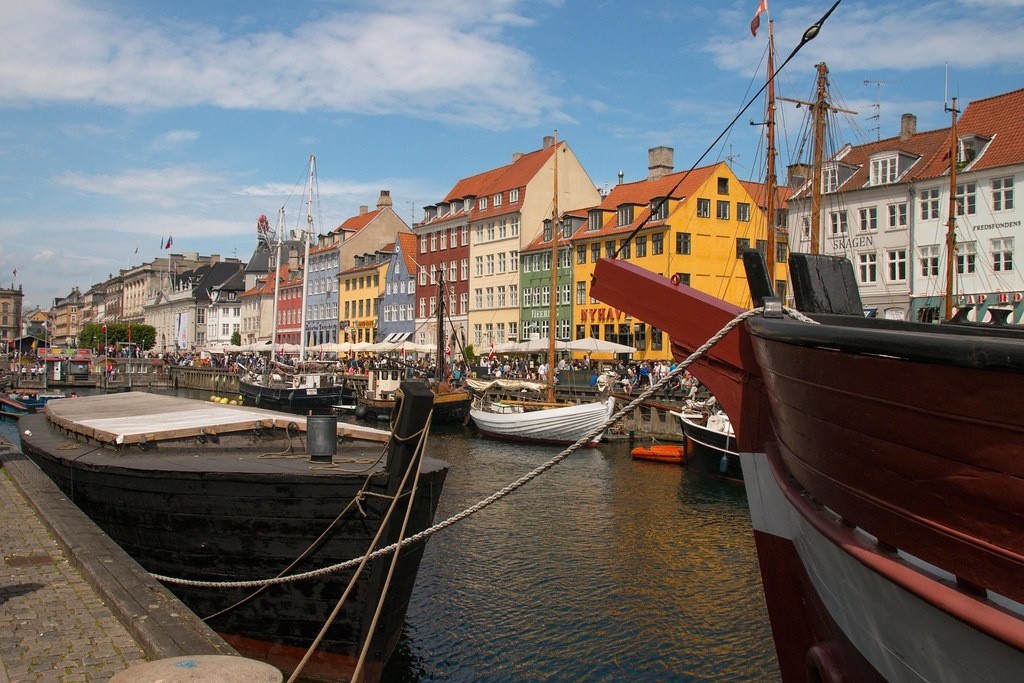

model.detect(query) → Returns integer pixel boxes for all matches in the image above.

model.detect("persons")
[6,346,701,403]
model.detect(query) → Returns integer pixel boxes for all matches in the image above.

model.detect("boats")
[0,2,1024,683]
